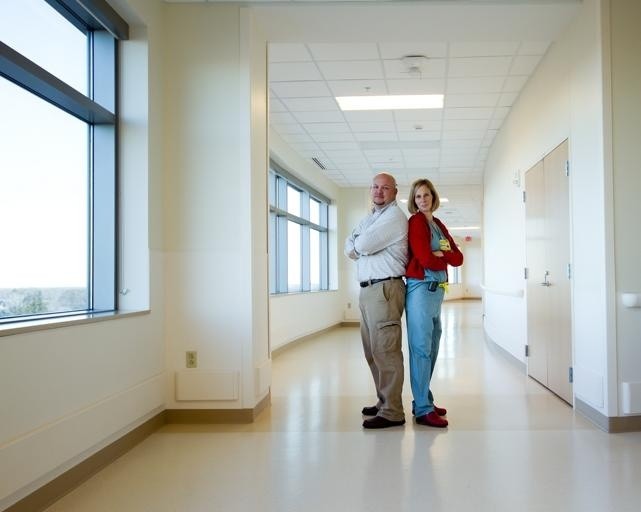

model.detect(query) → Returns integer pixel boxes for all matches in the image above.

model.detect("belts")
[360,276,402,287]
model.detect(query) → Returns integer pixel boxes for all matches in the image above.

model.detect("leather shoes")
[363,416,405,428]
[416,410,448,427]
[412,405,446,416]
[362,406,379,416]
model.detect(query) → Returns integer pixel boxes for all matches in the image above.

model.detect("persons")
[342,171,410,432]
[405,178,464,430]
